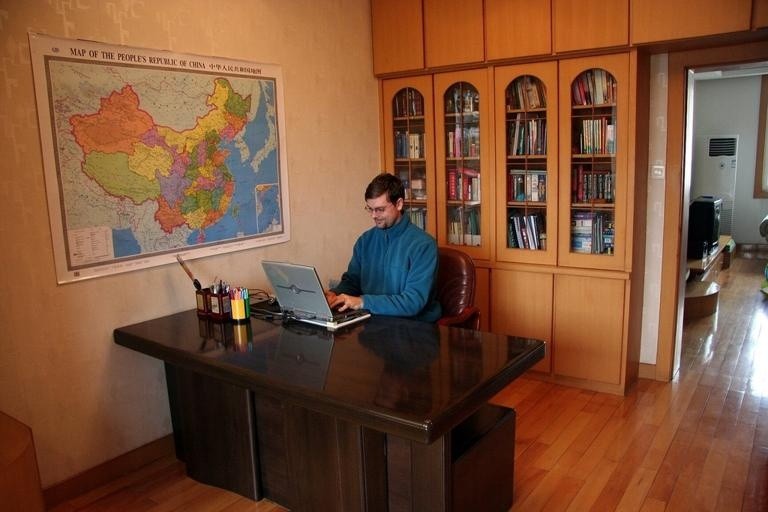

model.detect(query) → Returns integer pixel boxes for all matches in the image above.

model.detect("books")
[393,87,426,159]
[504,74,547,156]
[397,171,427,230]
[446,167,481,246]
[445,82,480,157]
[570,69,617,155]
[570,163,616,256]
[508,169,547,250]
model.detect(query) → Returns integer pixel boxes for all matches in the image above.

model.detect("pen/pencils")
[210,276,249,300]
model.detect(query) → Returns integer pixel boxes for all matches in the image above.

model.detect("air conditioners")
[694,131,740,235]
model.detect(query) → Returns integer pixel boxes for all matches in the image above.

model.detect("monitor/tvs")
[689,198,723,256]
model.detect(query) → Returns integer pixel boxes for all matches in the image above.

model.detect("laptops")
[262,260,370,322]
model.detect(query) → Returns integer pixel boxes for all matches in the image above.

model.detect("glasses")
[364,203,398,214]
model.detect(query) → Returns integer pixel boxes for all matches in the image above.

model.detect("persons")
[320,173,442,323]
[360,313,439,411]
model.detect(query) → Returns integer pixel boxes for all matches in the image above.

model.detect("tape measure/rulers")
[177,255,195,281]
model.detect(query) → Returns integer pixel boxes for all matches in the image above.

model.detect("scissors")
[194,279,204,293]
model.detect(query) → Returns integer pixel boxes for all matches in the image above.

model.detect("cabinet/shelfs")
[685,238,736,319]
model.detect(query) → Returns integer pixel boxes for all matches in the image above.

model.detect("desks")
[113,294,547,511]
